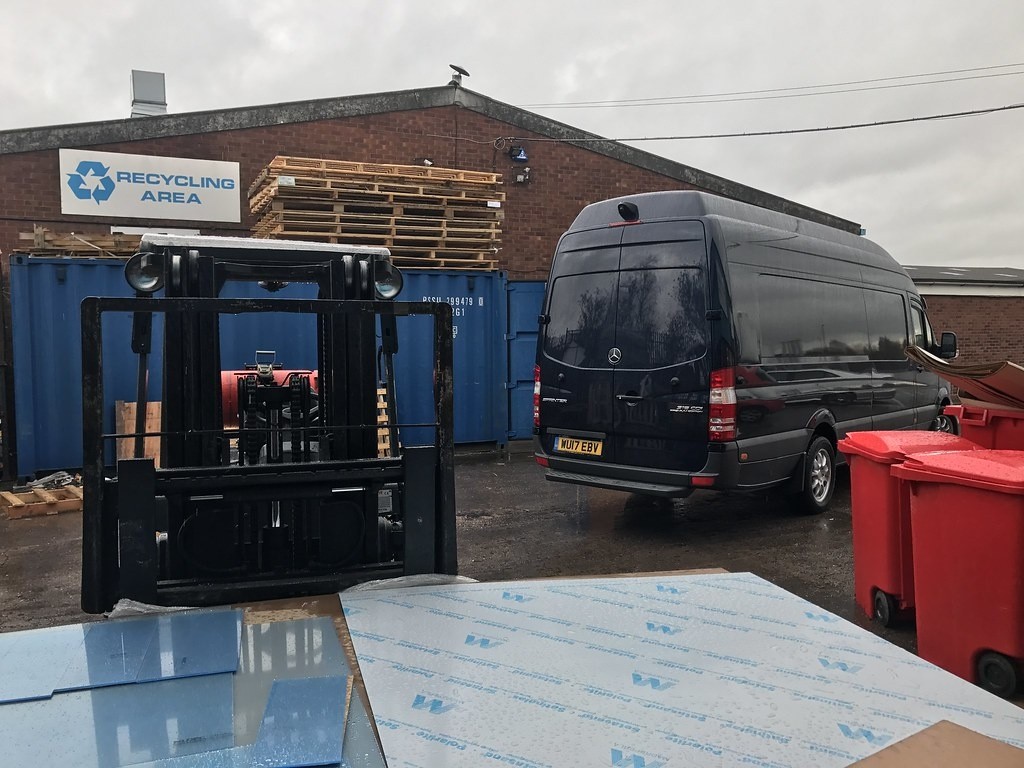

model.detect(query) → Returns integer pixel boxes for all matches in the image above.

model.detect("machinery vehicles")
[79,232,457,626]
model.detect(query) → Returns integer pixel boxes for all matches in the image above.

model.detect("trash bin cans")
[891,449,1024,700]
[837,431,991,627]
[943,404,1024,450]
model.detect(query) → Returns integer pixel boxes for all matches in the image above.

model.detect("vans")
[531,188,959,514]
